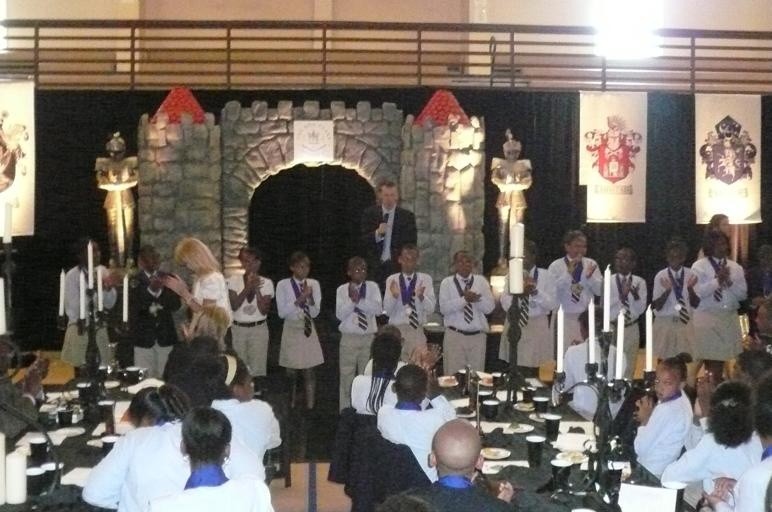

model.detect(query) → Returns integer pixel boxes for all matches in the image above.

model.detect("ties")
[518,271,539,330]
[564,256,584,304]
[668,267,691,326]
[614,273,633,326]
[289,278,314,338]
[452,276,477,324]
[707,256,727,301]
[350,283,370,332]
[399,276,419,331]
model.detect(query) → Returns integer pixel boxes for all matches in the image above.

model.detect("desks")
[0,357,153,512]
[422,363,700,511]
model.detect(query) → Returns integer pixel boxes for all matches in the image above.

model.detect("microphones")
[381,210,389,242]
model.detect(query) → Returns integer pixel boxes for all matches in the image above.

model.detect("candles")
[614,310,625,377]
[87,240,95,289]
[59,268,67,317]
[79,269,86,318]
[644,304,653,370]
[556,301,566,369]
[97,267,104,311]
[602,263,610,330]
[585,298,596,362]
[122,274,130,323]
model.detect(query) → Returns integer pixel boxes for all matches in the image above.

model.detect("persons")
[492,129,533,271]
[357,183,417,298]
[94,133,138,269]
[351,325,772,512]
[61,214,747,416]
[82,338,283,512]
[0,334,50,439]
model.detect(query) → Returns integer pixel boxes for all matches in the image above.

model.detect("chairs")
[251,367,428,509]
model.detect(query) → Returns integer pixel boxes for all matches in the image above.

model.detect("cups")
[436,368,590,493]
[6,453,27,505]
[25,360,146,503]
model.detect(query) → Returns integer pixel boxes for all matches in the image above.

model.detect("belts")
[448,325,481,337]
[231,318,266,328]
[609,319,639,328]
